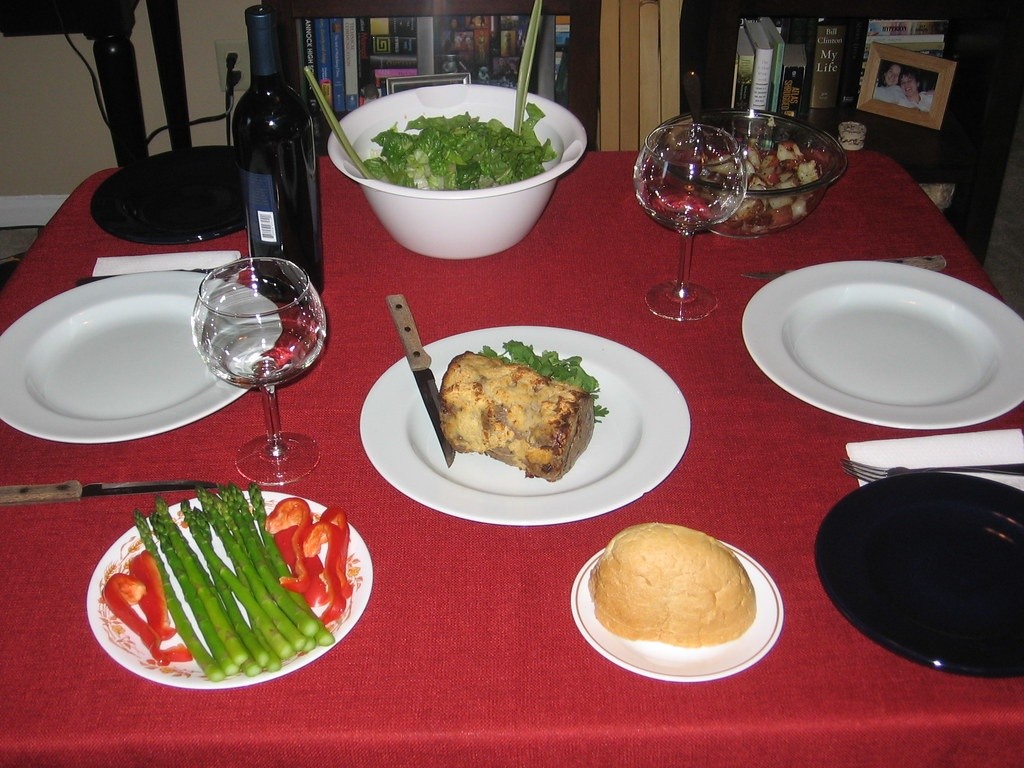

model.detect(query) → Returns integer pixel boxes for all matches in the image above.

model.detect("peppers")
[102,550,194,665]
[265,497,357,627]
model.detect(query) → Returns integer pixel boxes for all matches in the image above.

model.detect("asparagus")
[133,480,336,683]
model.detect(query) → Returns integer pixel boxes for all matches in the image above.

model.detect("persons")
[876,63,934,112]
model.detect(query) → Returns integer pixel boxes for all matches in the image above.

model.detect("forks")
[840,456,1024,483]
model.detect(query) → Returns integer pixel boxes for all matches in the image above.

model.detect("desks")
[1,150,1024,767]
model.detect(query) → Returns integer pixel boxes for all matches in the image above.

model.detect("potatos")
[705,138,821,232]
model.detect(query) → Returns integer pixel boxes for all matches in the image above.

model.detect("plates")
[571,535,782,682]
[0,270,281,443]
[815,472,1024,679]
[87,490,372,688]
[359,327,691,525]
[742,261,1024,430]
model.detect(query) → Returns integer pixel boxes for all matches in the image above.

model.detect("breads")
[439,351,596,482]
[589,521,756,650]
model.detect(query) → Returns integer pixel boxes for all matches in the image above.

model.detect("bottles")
[234,6,323,304]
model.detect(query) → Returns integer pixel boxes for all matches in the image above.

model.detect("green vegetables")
[478,340,610,425]
[364,102,557,192]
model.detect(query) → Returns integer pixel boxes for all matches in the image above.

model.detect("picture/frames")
[386,71,474,96]
[858,45,955,129]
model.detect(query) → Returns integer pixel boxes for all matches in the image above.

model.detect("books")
[303,17,417,112]
[732,20,845,118]
[857,19,945,85]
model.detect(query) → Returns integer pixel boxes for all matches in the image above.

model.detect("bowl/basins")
[327,84,586,258]
[649,111,847,238]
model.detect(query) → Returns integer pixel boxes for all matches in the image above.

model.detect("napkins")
[844,428,1024,487]
[91,251,242,281]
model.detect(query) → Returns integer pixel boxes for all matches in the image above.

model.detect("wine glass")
[632,123,746,322]
[193,257,330,482]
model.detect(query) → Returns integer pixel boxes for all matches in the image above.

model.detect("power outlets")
[215,39,251,92]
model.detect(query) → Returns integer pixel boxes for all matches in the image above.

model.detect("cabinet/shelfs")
[685,0,1024,262]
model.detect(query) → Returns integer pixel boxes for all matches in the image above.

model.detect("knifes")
[0,477,218,504]
[741,255,945,279]
[386,292,455,467]
